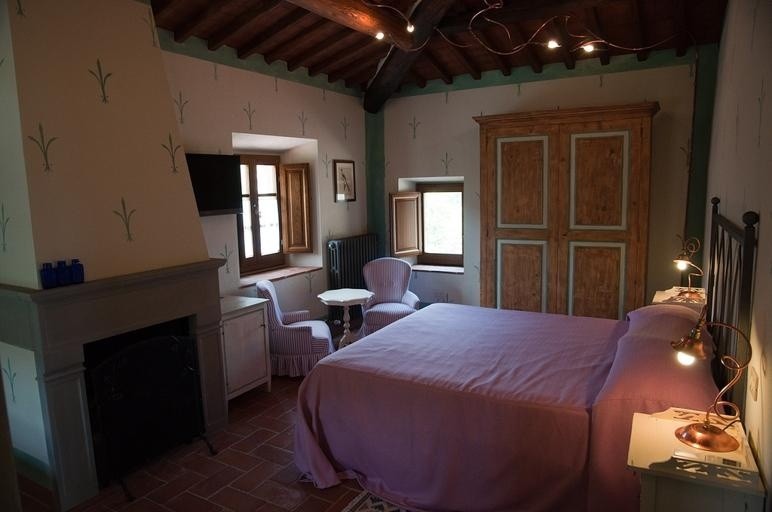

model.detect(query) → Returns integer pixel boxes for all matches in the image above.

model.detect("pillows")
[590,302,723,419]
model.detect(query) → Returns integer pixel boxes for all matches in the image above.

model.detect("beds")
[310,193,761,512]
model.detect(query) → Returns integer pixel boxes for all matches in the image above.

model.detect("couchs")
[361,258,421,337]
[252,279,335,378]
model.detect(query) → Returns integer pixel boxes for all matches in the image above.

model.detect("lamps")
[668,304,753,451]
[662,232,705,304]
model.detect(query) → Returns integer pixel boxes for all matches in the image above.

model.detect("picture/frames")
[332,158,357,202]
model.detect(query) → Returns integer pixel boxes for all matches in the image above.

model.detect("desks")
[316,287,376,351]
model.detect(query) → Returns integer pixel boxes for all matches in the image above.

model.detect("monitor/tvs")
[184,154,242,216]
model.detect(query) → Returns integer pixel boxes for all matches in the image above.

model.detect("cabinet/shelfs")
[471,100,662,321]
[219,293,272,417]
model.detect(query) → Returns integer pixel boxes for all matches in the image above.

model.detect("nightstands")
[651,286,706,309]
[624,407,766,512]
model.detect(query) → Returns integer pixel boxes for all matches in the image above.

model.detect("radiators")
[326,233,381,322]
